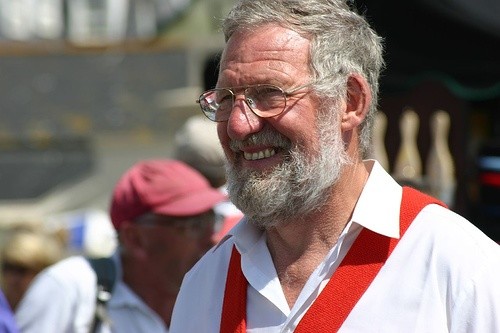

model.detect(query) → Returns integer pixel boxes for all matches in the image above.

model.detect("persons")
[0,114,243,333]
[167,0,500,333]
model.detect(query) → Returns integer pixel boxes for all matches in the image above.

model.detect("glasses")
[196,75,321,123]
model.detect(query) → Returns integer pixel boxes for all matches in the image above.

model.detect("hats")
[109,158,230,228]
